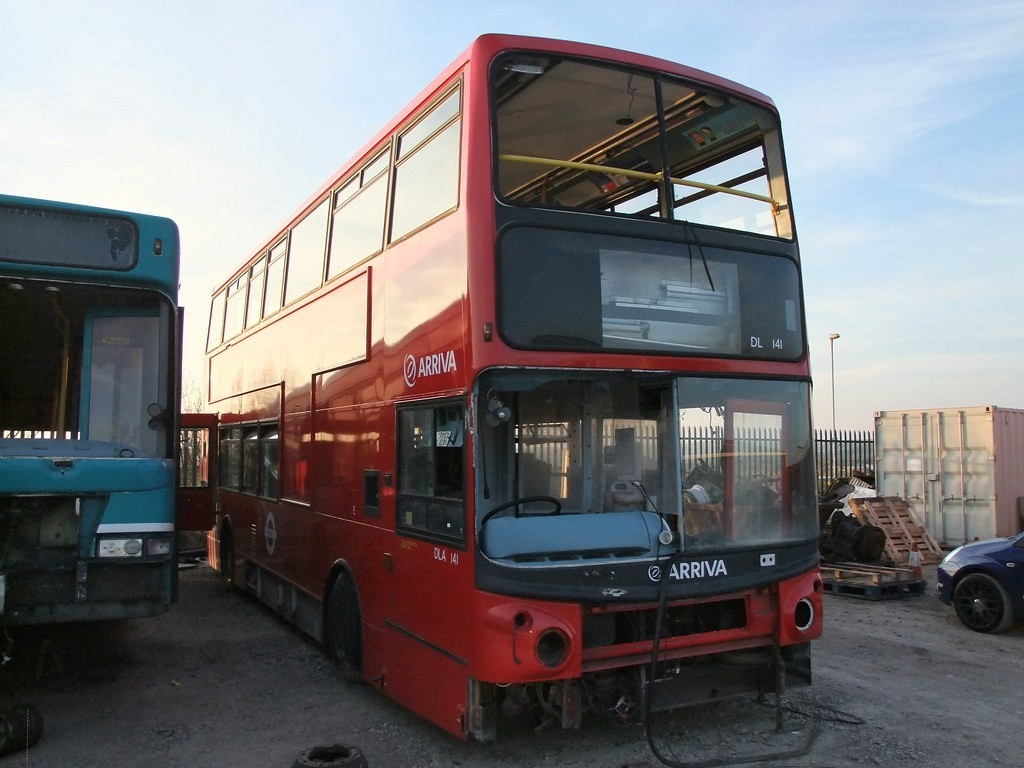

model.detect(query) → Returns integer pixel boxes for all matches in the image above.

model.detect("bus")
[179,33,864,767]
[0,194,181,629]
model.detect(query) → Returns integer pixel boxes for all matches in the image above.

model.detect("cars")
[937,531,1024,634]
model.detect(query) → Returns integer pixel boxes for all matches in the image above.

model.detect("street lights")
[828,333,840,481]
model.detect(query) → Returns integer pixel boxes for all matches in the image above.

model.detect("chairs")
[410,447,553,508]
